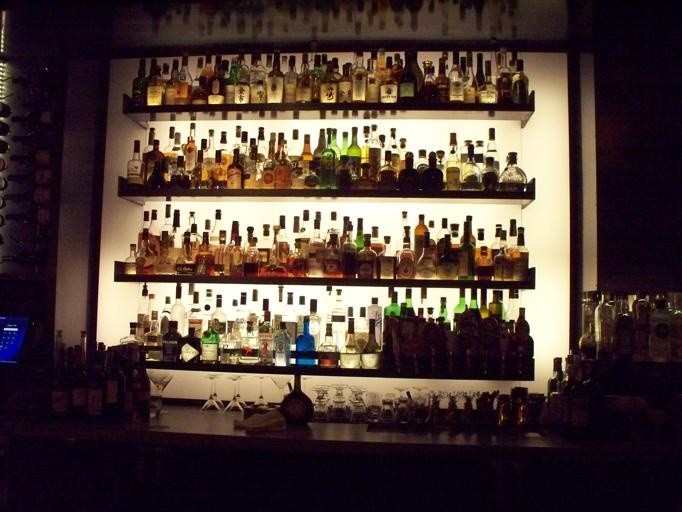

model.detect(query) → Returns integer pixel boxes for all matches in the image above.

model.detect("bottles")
[127,123,530,192]
[49,329,150,424]
[281,371,313,424]
[543,351,584,432]
[578,294,682,363]
[3,110,53,269]
[120,283,533,381]
[123,203,530,283]
[477,385,543,428]
[132,50,532,111]
[1,99,13,229]
[147,1,520,46]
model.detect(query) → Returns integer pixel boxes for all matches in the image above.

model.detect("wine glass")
[147,368,173,418]
[314,384,477,436]
[201,370,294,419]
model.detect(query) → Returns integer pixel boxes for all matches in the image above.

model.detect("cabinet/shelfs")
[114,39,536,381]
